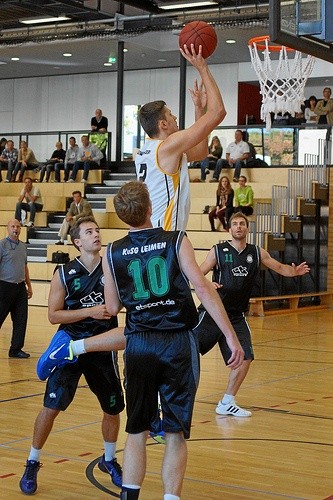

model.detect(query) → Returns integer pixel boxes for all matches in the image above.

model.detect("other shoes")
[81,179,86,183]
[66,179,75,183]
[25,221,34,228]
[233,177,239,182]
[42,179,47,182]
[55,241,64,245]
[205,168,210,174]
[4,179,9,182]
[9,347,30,358]
[36,179,41,182]
[209,178,218,182]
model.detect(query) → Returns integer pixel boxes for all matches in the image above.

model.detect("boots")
[10,174,16,182]
[19,174,23,182]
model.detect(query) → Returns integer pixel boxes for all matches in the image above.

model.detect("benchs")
[0,167,331,315]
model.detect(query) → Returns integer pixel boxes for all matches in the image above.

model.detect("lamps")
[157,0,218,10]
[19,12,72,24]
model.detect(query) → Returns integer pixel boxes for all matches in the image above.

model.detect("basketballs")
[179,21,218,61]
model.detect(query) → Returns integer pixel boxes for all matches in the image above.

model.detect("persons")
[209,130,250,182]
[15,178,43,228]
[36,141,66,183]
[55,190,95,245]
[0,135,39,183]
[102,181,244,500]
[19,216,123,495]
[313,88,333,124]
[304,96,317,123]
[193,212,309,417]
[194,136,223,182]
[233,176,254,216]
[242,131,257,161]
[208,176,234,232]
[0,219,32,358]
[51,137,80,183]
[37,44,227,433]
[67,135,104,183]
[90,109,108,166]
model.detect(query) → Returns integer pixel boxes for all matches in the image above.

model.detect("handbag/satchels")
[52,250,70,263]
[202,204,215,214]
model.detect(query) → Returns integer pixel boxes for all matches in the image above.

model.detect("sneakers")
[151,421,166,445]
[18,457,44,494]
[36,329,78,382]
[98,453,123,487]
[215,399,252,416]
